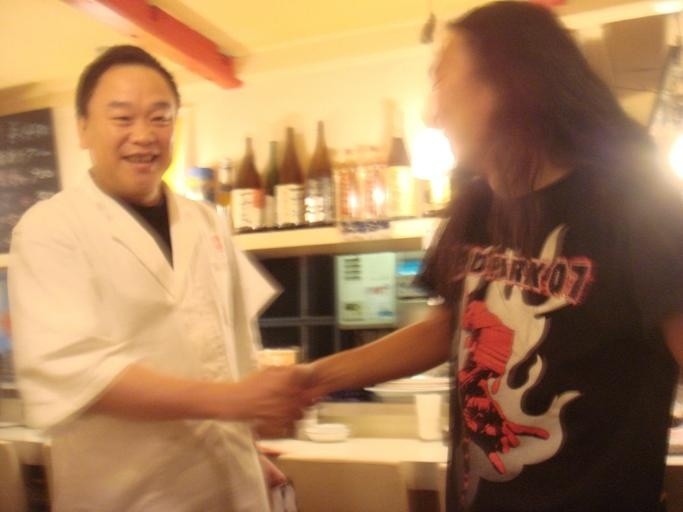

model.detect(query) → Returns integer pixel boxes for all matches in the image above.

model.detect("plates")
[365,376,456,401]
[303,424,352,442]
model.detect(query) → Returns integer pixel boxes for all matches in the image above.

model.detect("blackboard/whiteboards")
[0,108,61,253]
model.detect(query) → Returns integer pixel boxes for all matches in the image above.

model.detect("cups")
[417,393,441,443]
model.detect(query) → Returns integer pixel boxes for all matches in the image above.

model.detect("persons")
[284,0,682,512]
[5,44,313,512]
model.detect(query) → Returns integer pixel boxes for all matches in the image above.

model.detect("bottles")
[176,117,416,233]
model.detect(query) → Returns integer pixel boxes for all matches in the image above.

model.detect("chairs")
[0,440,30,512]
[276,453,410,512]
